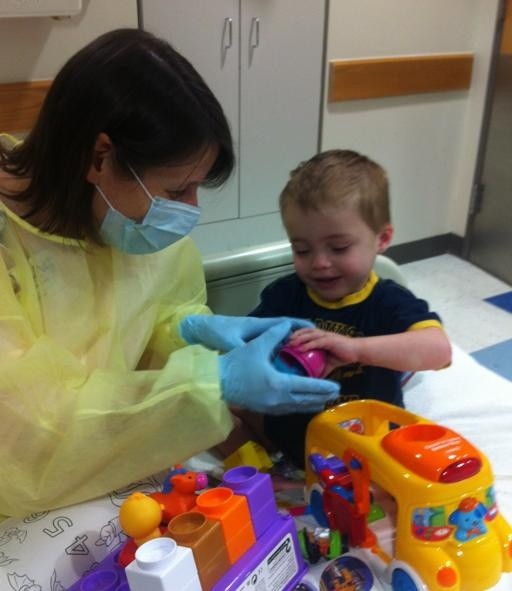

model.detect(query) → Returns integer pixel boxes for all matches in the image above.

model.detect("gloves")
[179,314,318,358]
[217,318,342,418]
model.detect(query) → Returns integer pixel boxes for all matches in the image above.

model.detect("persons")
[0,27,343,522]
[212,148,452,476]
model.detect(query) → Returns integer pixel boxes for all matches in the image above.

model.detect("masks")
[92,162,203,258]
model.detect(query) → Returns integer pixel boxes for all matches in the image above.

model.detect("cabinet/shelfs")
[141,1,326,226]
